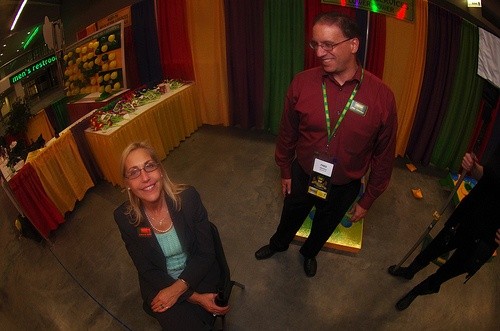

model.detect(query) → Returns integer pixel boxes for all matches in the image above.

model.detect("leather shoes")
[254,242,289,260]
[301,247,317,277]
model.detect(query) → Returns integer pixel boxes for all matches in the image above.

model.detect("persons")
[113,142,232,331]
[253,9,397,277]
[388,140,500,310]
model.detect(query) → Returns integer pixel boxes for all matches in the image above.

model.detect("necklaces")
[143,207,170,225]
[141,207,174,233]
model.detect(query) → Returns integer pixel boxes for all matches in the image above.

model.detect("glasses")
[308,36,355,52]
[123,160,160,180]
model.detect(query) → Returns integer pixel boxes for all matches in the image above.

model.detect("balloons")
[64,34,120,96]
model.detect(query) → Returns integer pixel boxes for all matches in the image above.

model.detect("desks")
[84,82,203,193]
[65,87,131,153]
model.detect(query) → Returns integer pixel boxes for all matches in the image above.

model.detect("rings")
[213,313,216,316]
[160,304,164,308]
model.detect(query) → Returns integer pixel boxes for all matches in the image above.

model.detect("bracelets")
[178,278,190,286]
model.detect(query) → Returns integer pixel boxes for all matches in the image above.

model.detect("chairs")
[143,220,246,331]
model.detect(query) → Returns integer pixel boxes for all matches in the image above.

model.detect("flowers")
[90,79,190,131]
[13,216,28,241]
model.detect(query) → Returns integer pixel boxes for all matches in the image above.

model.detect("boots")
[395,273,441,311]
[387,254,430,280]
